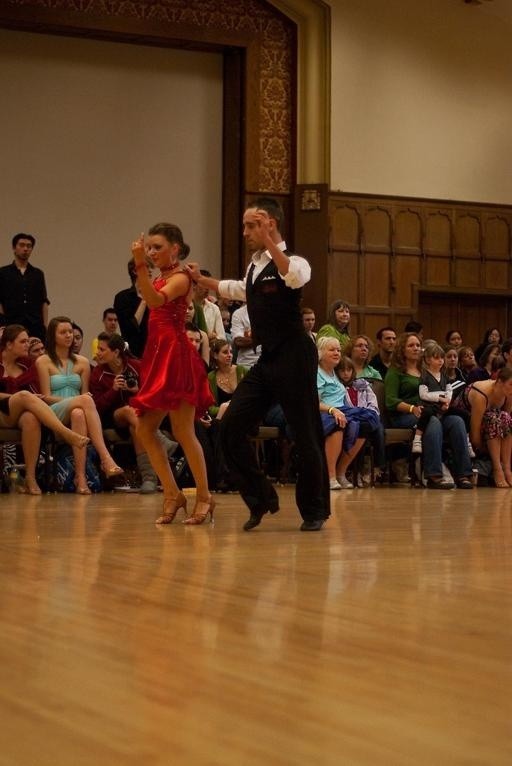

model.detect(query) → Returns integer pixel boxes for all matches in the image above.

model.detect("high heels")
[100,459,123,478]
[492,471,510,488]
[181,496,216,524]
[78,435,89,449]
[154,491,187,524]
[23,477,42,495]
[73,477,91,495]
[506,479,512,487]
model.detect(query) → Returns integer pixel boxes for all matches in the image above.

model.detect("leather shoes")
[329,479,341,490]
[300,518,325,532]
[242,499,279,531]
[340,478,354,489]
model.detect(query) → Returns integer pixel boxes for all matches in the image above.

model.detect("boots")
[157,429,178,457]
[136,451,158,493]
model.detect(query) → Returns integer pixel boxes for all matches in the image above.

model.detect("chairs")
[349,376,420,489]
[0,417,283,494]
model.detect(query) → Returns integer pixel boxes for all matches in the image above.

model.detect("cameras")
[122,372,138,388]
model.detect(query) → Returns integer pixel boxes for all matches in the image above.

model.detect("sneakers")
[427,478,454,489]
[467,442,476,457]
[455,479,473,489]
[411,440,422,453]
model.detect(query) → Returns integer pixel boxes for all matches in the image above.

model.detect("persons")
[184,197,332,533]
[1,232,50,346]
[126,221,218,525]
[1,256,512,496]
